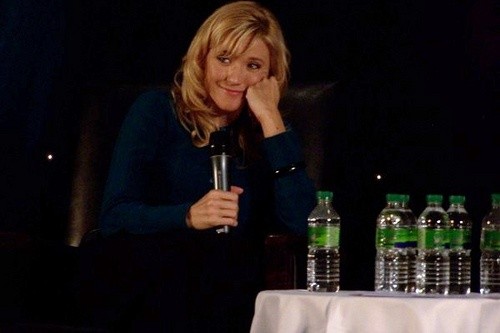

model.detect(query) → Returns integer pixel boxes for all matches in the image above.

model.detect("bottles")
[480,192,500,295]
[307,191,340,293]
[417,195,452,294]
[376,194,408,293]
[398,194,418,293]
[446,196,471,295]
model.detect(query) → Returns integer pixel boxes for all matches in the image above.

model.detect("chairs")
[64,81,336,333]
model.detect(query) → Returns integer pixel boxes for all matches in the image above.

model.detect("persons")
[95,1,319,333]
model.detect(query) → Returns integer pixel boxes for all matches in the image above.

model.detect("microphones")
[209,131,233,234]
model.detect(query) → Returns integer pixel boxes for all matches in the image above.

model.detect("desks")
[250,289,500,333]
